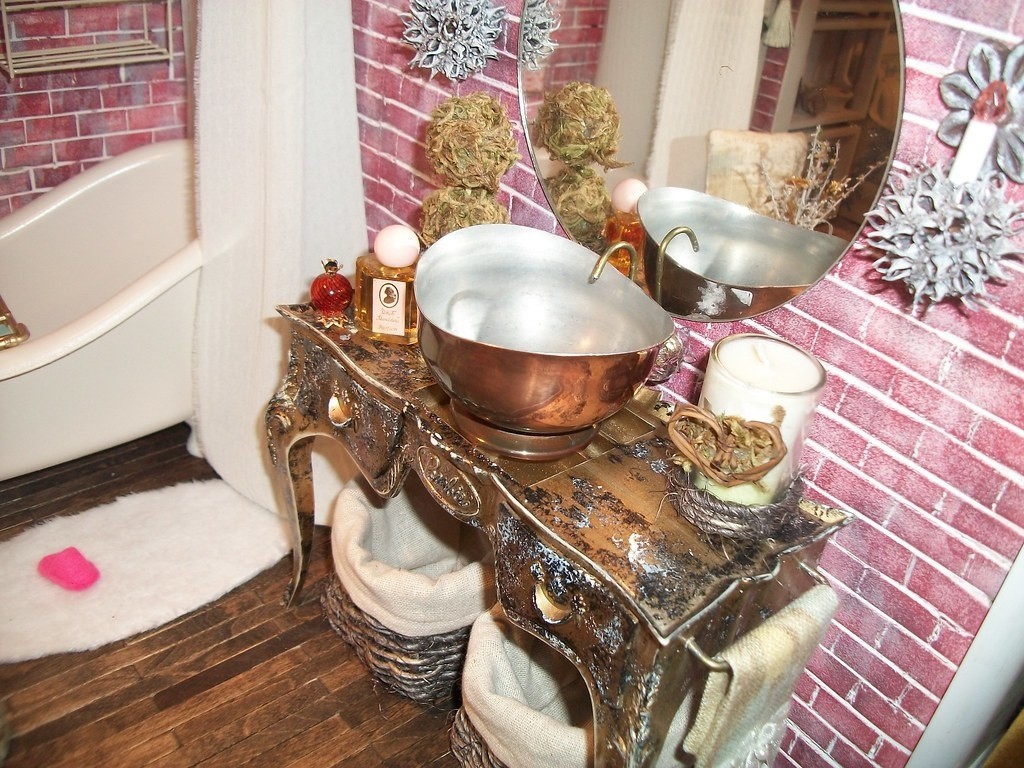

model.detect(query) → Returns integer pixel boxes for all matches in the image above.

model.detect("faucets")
[587,240,639,283]
[657,225,699,303]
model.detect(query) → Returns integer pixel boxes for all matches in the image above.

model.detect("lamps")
[853,38,1024,317]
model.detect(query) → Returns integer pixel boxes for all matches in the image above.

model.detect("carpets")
[0,479,293,664]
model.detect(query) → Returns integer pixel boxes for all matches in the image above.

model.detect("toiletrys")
[605,179,648,273]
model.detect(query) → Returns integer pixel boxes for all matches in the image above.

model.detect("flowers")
[753,124,890,230]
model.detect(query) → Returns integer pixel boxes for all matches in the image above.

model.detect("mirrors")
[519,0,905,323]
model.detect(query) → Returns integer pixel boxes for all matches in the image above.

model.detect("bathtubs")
[2,138,203,484]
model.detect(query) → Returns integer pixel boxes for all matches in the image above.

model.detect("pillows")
[707,128,809,221]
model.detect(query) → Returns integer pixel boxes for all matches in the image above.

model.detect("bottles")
[354,225,421,345]
[605,178,649,275]
[310,258,352,328]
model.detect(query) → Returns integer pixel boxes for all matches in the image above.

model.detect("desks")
[264,290,858,768]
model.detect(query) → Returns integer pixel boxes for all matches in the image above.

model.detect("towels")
[685,581,836,766]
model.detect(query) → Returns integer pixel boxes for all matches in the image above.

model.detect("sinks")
[415,223,675,462]
[637,187,851,322]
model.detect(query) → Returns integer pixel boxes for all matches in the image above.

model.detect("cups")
[684,332,827,509]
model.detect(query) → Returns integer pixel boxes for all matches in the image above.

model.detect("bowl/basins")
[411,223,679,437]
[635,184,852,320]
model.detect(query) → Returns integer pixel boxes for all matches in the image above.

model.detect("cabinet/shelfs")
[0,0,174,78]
[751,1,896,218]
